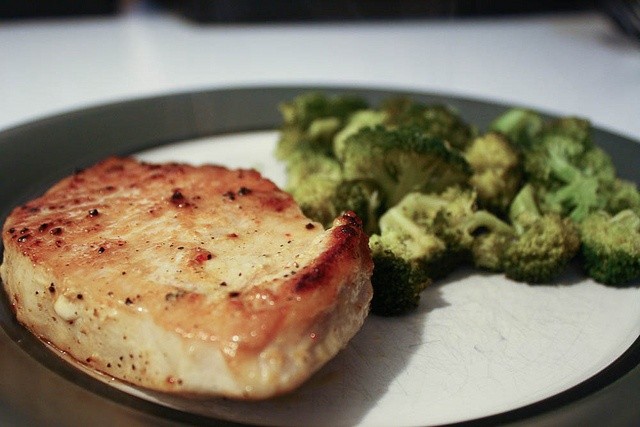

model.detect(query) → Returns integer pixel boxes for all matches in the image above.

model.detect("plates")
[34,119,639,426]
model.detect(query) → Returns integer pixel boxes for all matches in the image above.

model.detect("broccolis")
[274,92,639,318]
